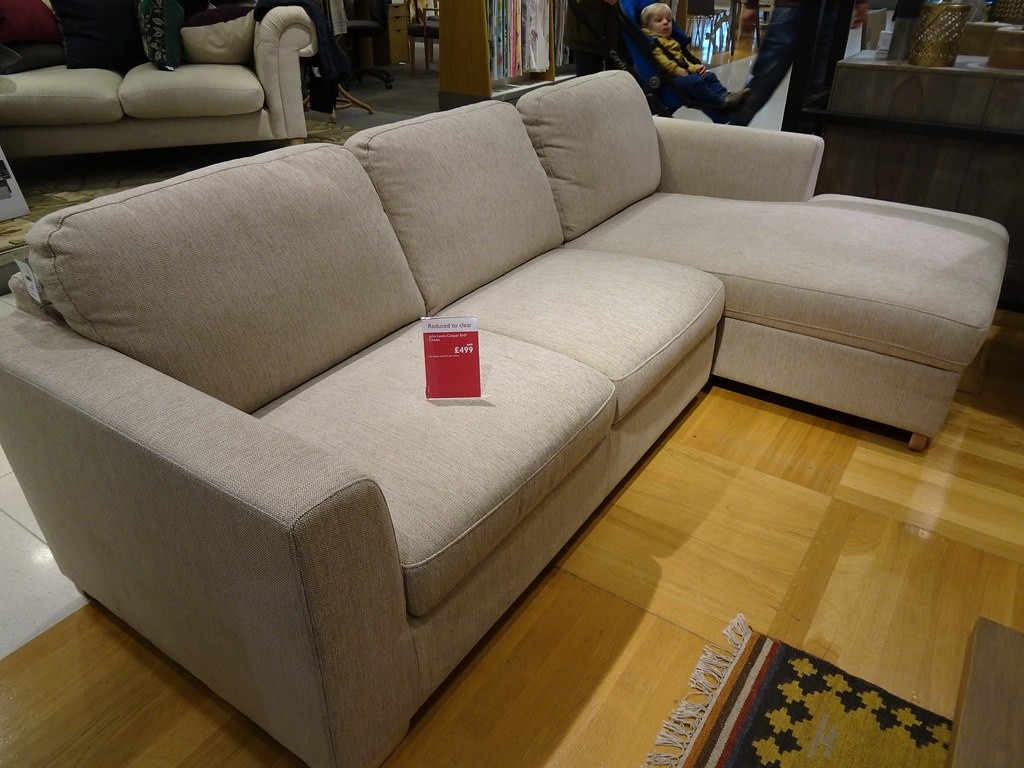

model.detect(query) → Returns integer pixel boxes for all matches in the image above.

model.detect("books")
[485,0,570,80]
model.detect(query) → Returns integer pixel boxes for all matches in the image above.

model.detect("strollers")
[613,0,687,119]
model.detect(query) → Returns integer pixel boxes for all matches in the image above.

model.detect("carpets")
[642,612,953,768]
[0,381,1024,768]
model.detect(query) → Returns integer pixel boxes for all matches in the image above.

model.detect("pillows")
[179,7,256,64]
[137,0,183,70]
[48,0,138,70]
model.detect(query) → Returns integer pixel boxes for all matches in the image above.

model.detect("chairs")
[300,77,373,124]
[687,0,729,57]
[335,19,395,88]
[410,8,440,67]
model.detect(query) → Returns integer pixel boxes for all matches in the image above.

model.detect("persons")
[729,1,870,126]
[640,3,753,108]
[564,0,632,78]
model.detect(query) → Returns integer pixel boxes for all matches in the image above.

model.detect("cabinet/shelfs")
[438,0,578,109]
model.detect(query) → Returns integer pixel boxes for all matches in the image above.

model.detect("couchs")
[0,0,319,174]
[0,66,1014,768]
